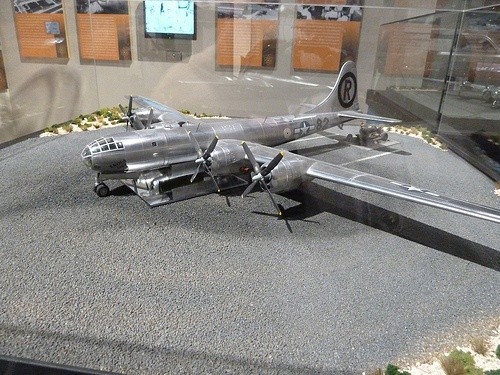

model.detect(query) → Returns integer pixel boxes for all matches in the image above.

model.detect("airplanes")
[79,60,500,243]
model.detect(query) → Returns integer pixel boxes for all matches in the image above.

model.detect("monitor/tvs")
[143,0,197,41]
